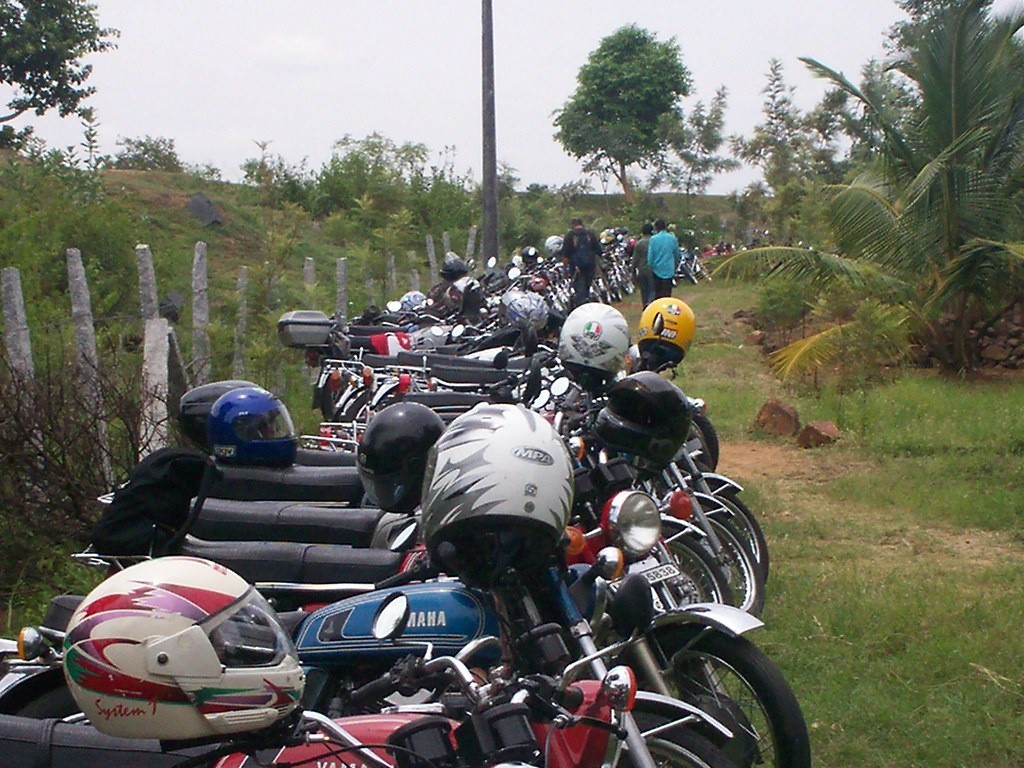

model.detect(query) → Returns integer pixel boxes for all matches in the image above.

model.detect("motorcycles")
[2,215,812,767]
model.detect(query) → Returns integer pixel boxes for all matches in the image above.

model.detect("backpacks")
[570,227,596,268]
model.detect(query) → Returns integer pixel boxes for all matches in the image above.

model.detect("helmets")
[60,555,307,739]
[420,402,576,550]
[544,235,564,260]
[557,302,630,373]
[439,252,468,282]
[503,293,550,331]
[592,369,692,464]
[399,291,427,313]
[444,276,480,312]
[521,246,539,264]
[204,388,299,467]
[635,297,697,355]
[354,401,448,513]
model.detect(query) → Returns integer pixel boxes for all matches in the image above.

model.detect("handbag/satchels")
[84,446,222,559]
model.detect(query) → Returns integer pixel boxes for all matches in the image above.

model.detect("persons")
[562,218,607,308]
[631,219,681,310]
